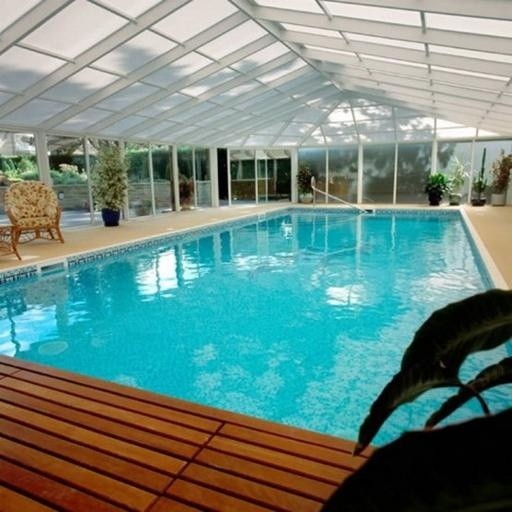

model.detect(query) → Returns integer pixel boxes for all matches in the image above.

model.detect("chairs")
[5,181,65,246]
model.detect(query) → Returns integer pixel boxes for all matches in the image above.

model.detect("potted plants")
[297,166,314,203]
[423,149,512,205]
[90,141,127,228]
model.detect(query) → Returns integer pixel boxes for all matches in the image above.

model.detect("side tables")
[0,224,22,261]
